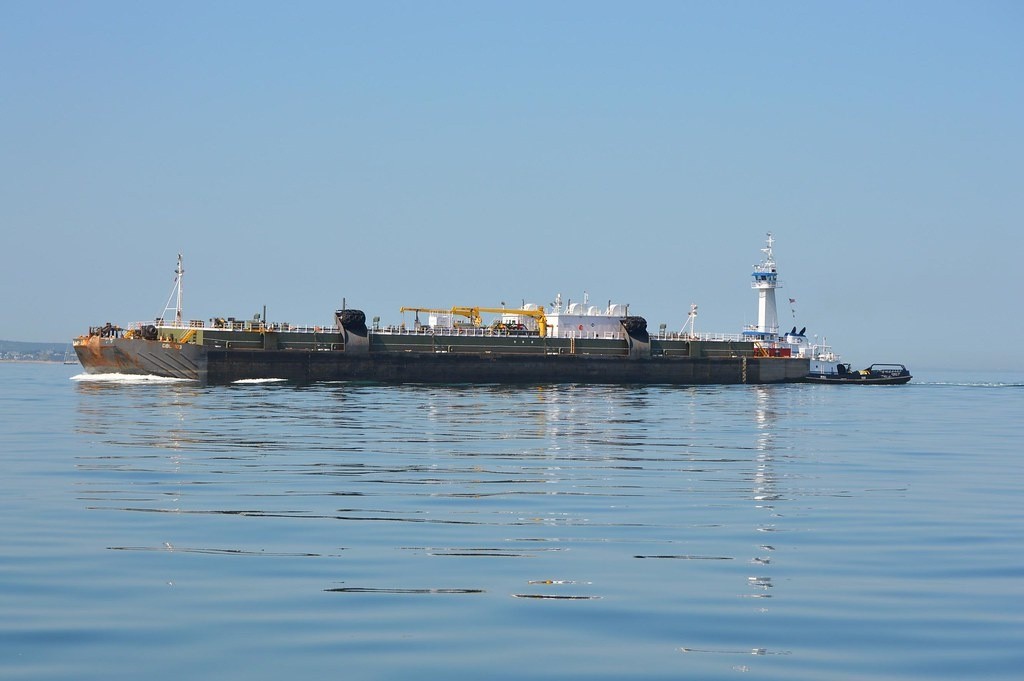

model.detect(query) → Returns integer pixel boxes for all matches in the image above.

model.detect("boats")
[806,362,914,384]
[69,232,842,386]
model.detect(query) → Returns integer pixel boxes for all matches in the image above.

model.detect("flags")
[790,298,795,302]
